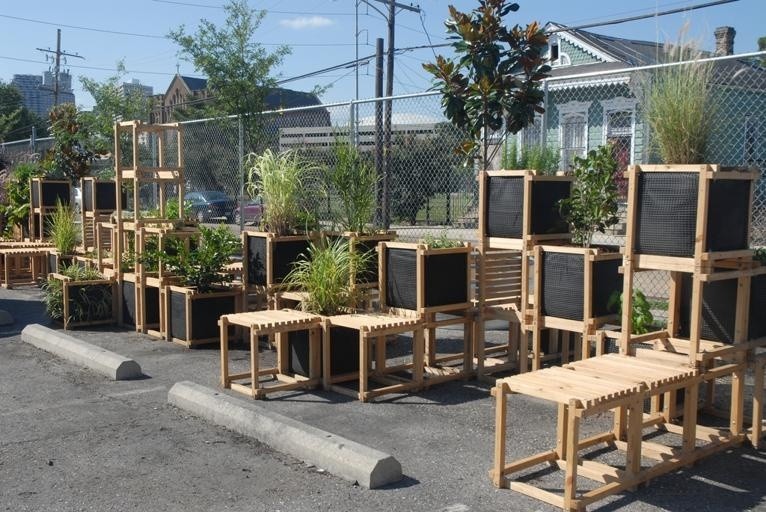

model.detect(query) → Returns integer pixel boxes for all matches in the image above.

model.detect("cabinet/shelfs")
[471,151,762,510]
[213,225,471,408]
[2,171,128,330]
[110,114,243,354]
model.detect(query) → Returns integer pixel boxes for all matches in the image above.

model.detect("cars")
[232,192,315,224]
[184,190,236,222]
